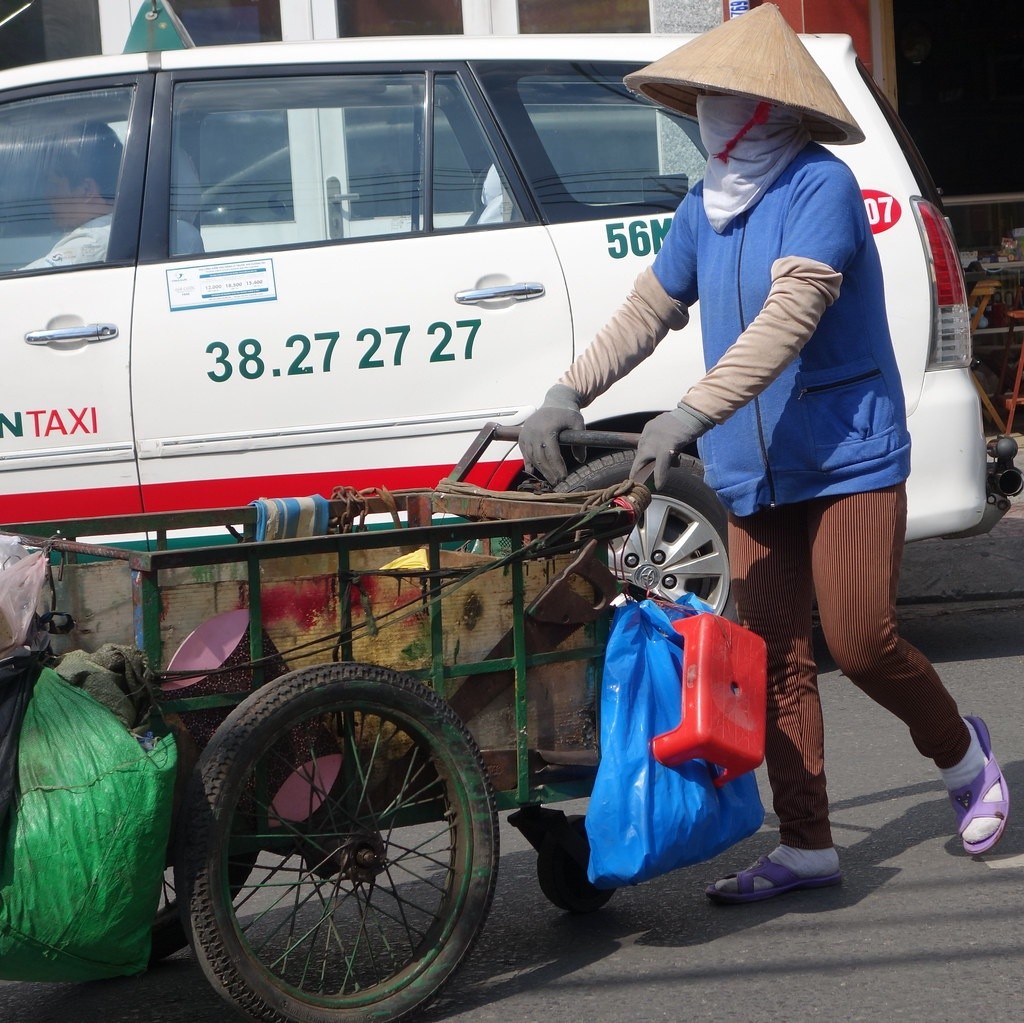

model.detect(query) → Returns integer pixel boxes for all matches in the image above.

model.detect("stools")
[651,612,767,788]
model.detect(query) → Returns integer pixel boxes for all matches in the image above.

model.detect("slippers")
[704,852,843,903]
[946,716,1010,855]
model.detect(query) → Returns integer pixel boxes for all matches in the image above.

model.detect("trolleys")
[0,421,733,1022]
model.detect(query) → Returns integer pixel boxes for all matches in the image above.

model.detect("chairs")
[463,162,513,226]
[171,147,205,255]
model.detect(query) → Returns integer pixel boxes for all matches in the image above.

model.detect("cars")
[0,26,998,865]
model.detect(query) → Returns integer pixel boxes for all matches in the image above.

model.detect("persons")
[517,2,1009,904]
[13,121,123,272]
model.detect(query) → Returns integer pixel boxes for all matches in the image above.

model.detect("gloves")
[629,403,715,492]
[518,385,590,486]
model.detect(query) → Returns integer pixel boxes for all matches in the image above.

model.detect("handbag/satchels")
[585,595,766,888]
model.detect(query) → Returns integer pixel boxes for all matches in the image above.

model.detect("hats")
[621,3,865,146]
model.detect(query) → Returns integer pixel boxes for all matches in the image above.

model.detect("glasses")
[39,177,62,202]
